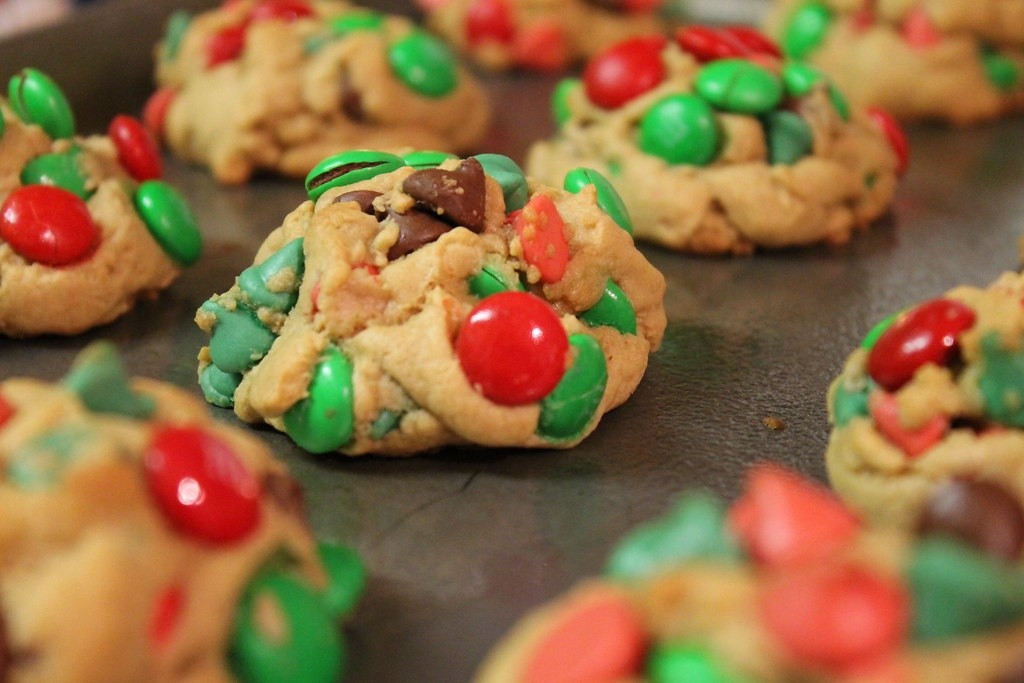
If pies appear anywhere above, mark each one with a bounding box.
[0,0,1024,683]
